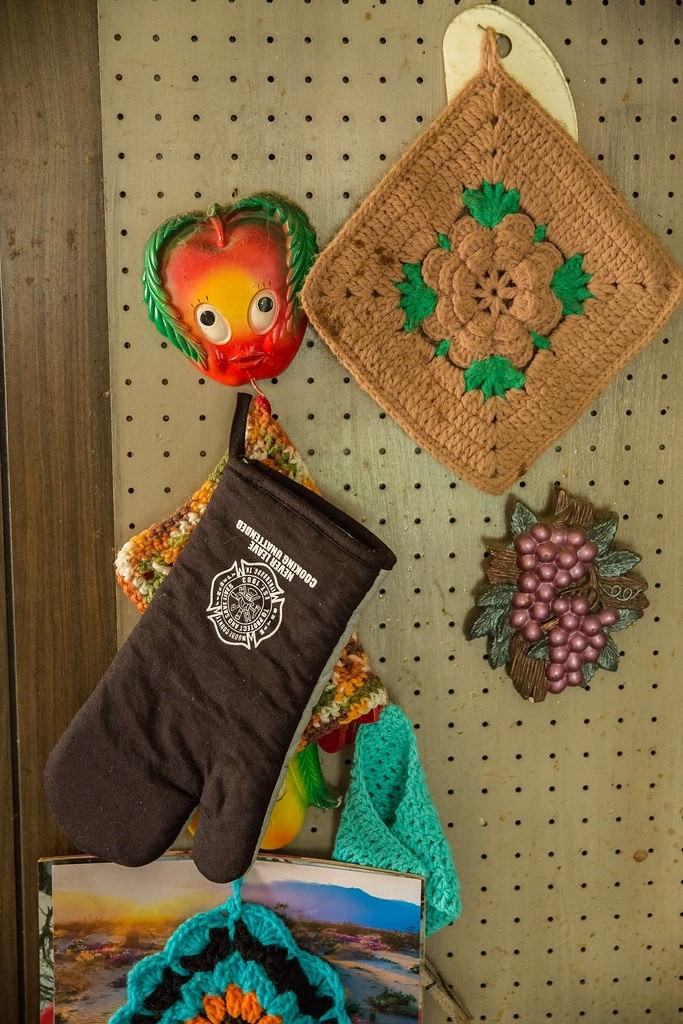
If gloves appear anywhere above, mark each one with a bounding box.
[40,455,398,884]
